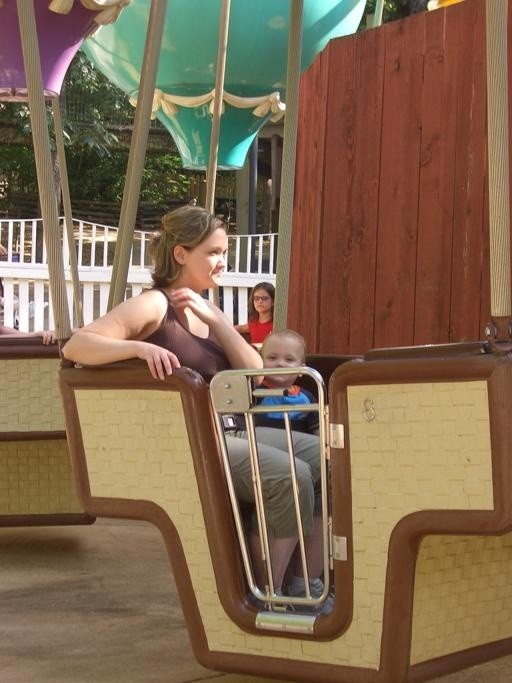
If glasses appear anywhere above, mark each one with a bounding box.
[254,295,272,301]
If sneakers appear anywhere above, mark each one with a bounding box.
[282,578,335,613]
[248,586,295,612]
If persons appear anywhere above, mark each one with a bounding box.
[63,206,340,615]
[0,322,79,345]
[256,328,323,435]
[232,281,276,347]
